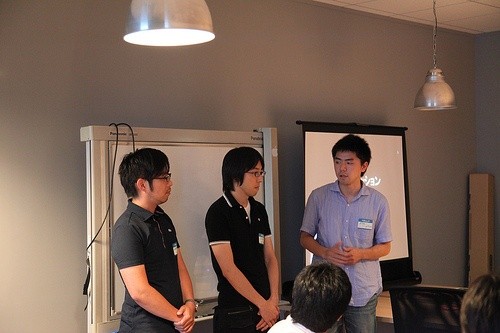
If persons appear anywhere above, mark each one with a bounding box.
[299,135,393,333]
[267,261,352,333]
[111,148,199,333]
[204,147,280,333]
[459,274,500,333]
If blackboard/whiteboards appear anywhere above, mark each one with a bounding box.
[81,125,285,333]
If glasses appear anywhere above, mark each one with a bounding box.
[153,173,172,181]
[246,171,266,177]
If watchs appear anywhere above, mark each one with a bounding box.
[185,299,199,312]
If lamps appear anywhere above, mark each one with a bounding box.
[413,0,459,112]
[122,0,215,47]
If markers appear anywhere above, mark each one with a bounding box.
[195,314,214,319]
